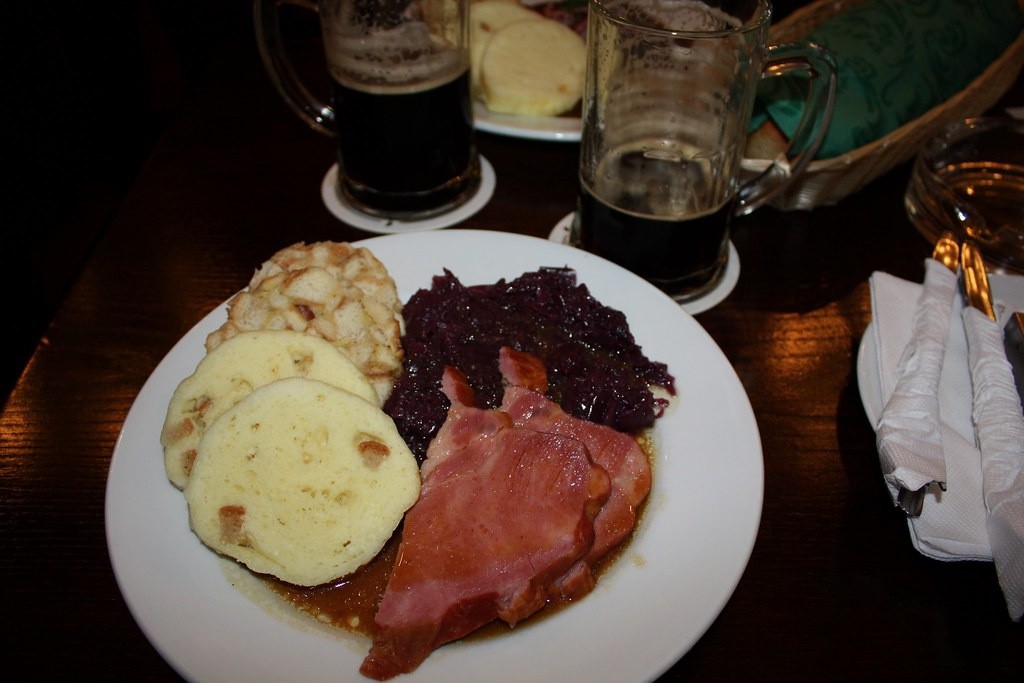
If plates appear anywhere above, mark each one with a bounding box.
[466,107,583,155]
[733,0,1024,208]
[104,228,766,683]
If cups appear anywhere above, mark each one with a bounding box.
[567,0,837,308]
[905,118,1024,319]
[248,0,484,220]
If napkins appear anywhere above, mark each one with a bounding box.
[868,272,994,562]
[961,305,1024,622]
[874,260,956,491]
